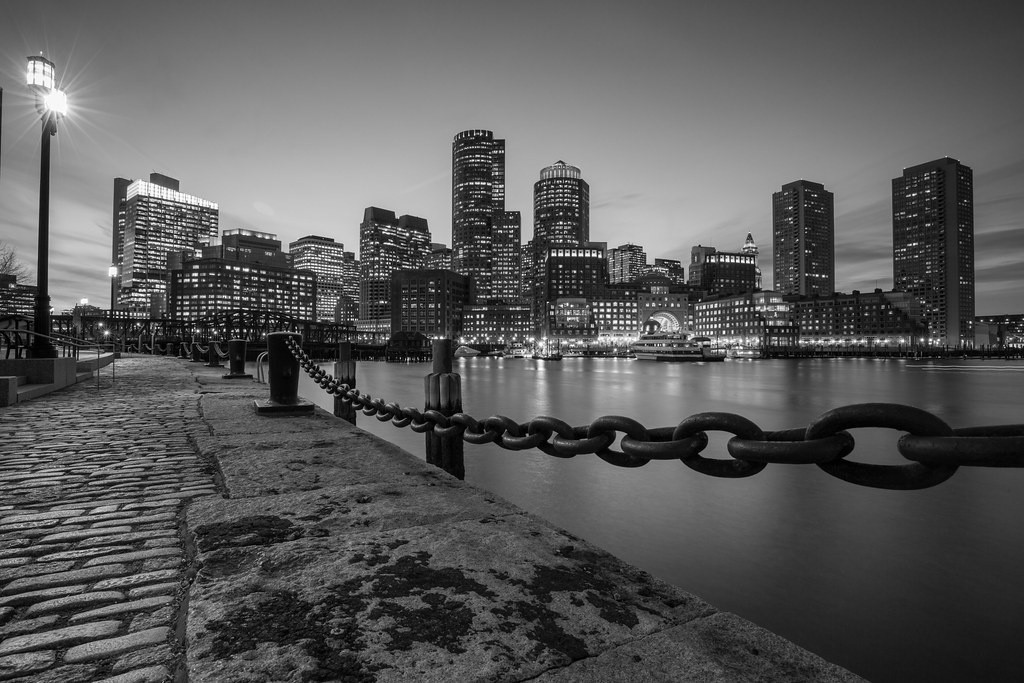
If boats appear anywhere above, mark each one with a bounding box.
[632,332,729,361]
[531,337,563,361]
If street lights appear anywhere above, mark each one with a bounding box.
[26,53,69,357]
